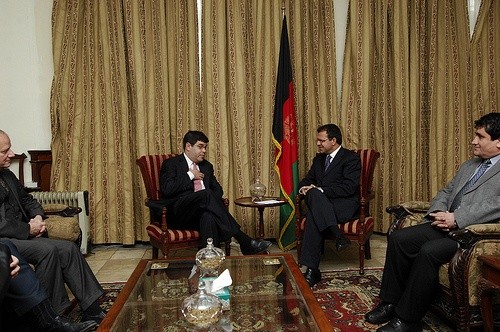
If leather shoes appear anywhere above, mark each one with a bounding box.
[241,239,272,251]
[304,267,322,286]
[82,310,107,322]
[53,316,97,332]
[376,320,423,332]
[336,237,351,250]
[365,301,400,324]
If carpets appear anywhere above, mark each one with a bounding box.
[63,267,459,332]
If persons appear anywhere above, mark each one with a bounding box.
[0,235,98,332]
[158,131,274,257]
[0,128,108,328]
[364,112,500,332]
[296,123,362,291]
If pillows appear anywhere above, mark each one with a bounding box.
[44,217,81,241]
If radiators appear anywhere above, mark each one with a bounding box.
[28,191,78,218]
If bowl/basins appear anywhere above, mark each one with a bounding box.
[194,237,227,273]
[181,280,224,329]
[249,177,267,201]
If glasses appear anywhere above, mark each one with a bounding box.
[319,138,330,144]
[193,144,208,150]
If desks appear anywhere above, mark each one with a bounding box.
[96,253,335,332]
[234,197,288,255]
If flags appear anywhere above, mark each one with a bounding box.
[273,14,304,253]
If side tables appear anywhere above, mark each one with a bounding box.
[477,254,500,332]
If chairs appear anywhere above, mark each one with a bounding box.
[386,202,500,332]
[296,149,389,270]
[9,153,27,187]
[136,154,230,259]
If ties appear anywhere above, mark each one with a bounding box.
[325,156,332,171]
[192,162,202,192]
[449,159,492,212]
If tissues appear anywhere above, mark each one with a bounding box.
[198,268,233,311]
